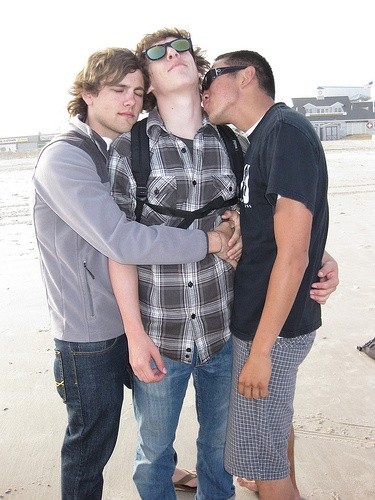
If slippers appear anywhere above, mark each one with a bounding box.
[174,469,198,493]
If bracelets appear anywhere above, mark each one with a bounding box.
[214,229,224,256]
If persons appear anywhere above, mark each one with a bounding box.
[201,50,330,500]
[30,48,243,499]
[107,28,339,500]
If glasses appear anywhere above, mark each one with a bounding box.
[141,38,191,61]
[202,65,246,95]
